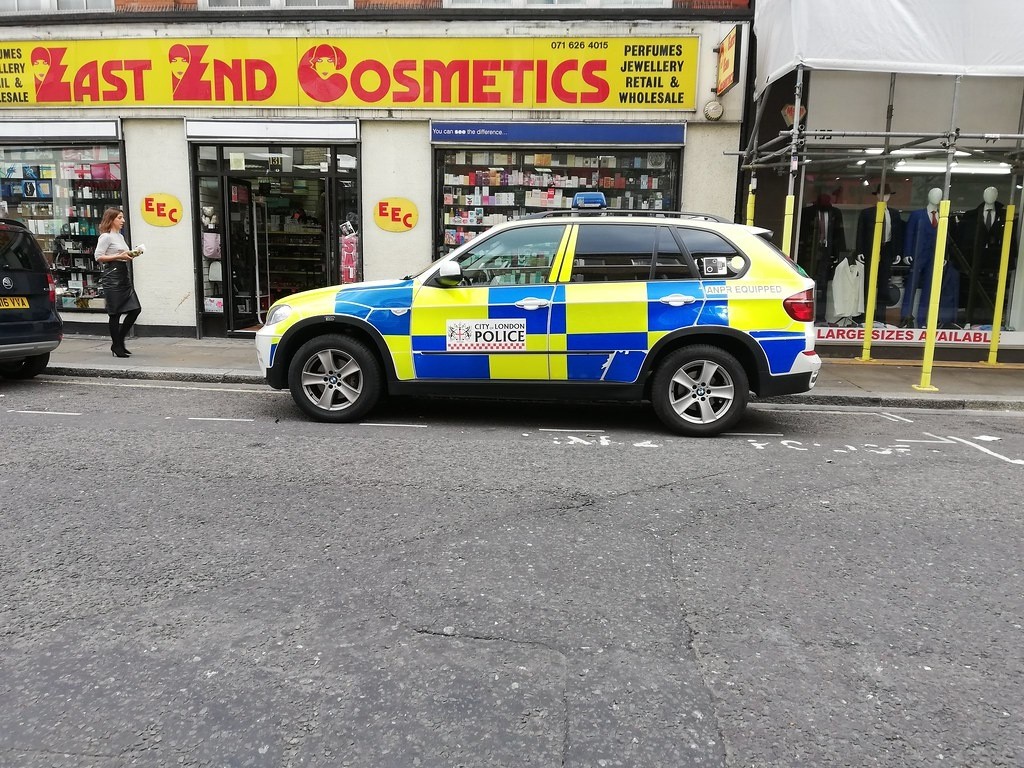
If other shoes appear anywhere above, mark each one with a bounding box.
[897,314,915,328]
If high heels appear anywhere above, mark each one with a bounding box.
[111,340,131,358]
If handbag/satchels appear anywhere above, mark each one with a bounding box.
[203,233,224,313]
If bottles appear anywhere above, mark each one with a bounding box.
[449,208,454,225]
[455,209,460,223]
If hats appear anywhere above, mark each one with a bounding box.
[813,193,832,211]
[872,184,896,195]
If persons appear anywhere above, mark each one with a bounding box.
[94,207,141,358]
[796,183,1017,329]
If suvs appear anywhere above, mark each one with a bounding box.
[255,192,824,438]
[0,217,63,386]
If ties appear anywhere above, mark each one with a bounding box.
[931,211,937,229]
[882,213,886,246]
[818,211,825,241]
[986,209,993,232]
